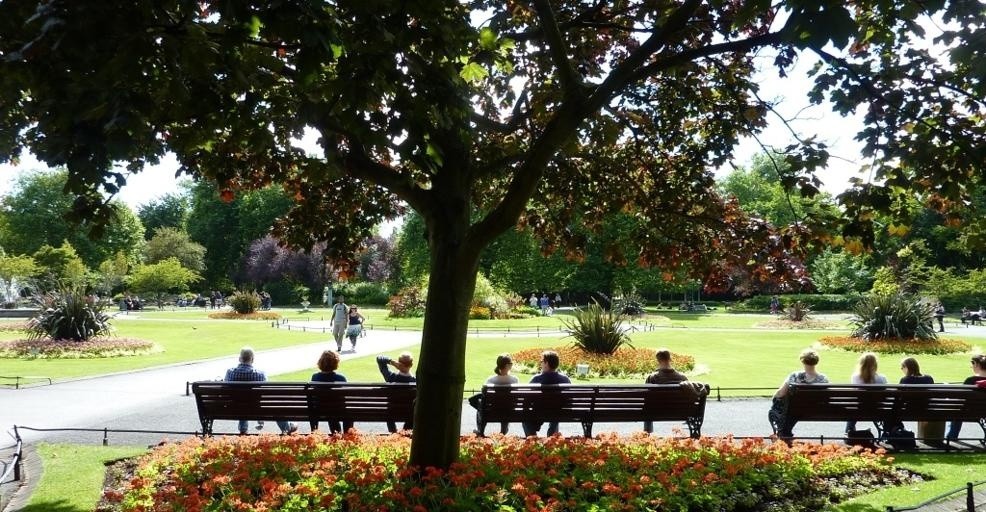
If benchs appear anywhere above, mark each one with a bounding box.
[190,377,414,439]
[765,379,986,446]
[474,383,708,441]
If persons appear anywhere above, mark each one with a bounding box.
[524,353,570,436]
[656,296,780,315]
[529,293,538,306]
[948,354,986,442]
[540,294,549,316]
[224,350,297,434]
[375,353,415,433]
[40,287,274,310]
[311,350,355,436]
[846,354,886,437]
[328,292,348,354]
[344,305,364,350]
[923,301,985,334]
[644,350,688,434]
[472,356,520,433]
[555,292,561,309]
[893,358,934,414]
[775,350,827,415]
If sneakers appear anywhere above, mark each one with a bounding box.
[282,422,297,435]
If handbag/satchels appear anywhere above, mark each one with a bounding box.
[885,430,916,450]
[848,429,876,452]
